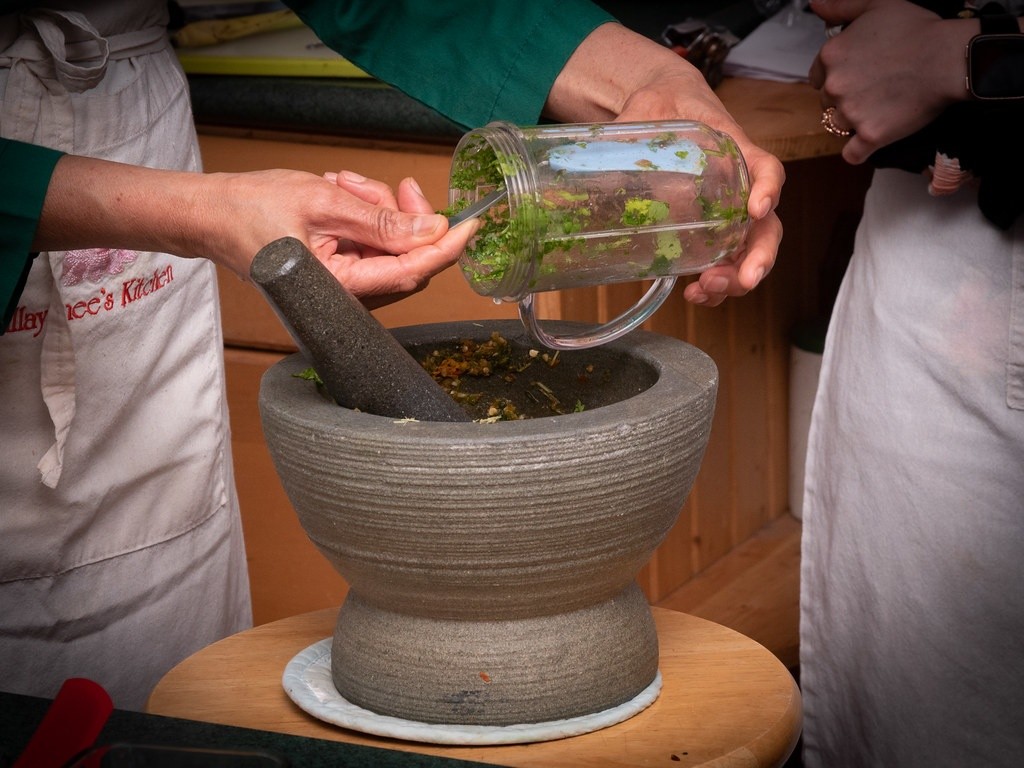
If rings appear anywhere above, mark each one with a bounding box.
[821,107,851,137]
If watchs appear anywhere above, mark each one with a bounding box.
[964,2,1024,123]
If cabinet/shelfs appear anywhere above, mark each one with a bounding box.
[202,76,841,658]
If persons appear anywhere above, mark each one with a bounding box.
[0,0,786,714]
[797,0,1024,768]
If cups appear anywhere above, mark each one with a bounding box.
[449,123,753,350]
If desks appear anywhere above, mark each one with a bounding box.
[145,600,804,768]
